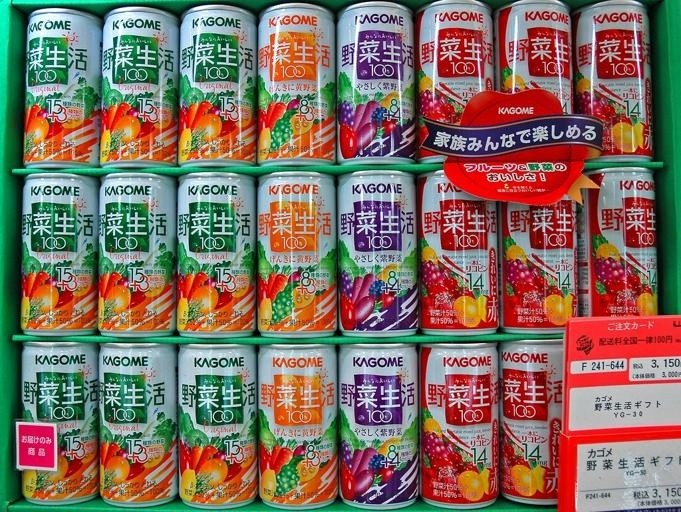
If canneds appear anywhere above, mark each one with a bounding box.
[176,2,258,172]
[500,340,565,507]
[178,345,258,510]
[421,343,501,510]
[17,342,100,507]
[258,3,337,165]
[99,6,179,169]
[22,8,102,170]
[100,343,179,508]
[418,172,499,334]
[18,174,99,337]
[258,170,338,340]
[500,193,579,334]
[178,172,258,338]
[573,3,656,164]
[495,0,577,119]
[417,1,497,165]
[100,171,178,338]
[579,167,659,319]
[337,344,421,511]
[338,170,419,336]
[337,1,418,167]
[258,345,337,511]
